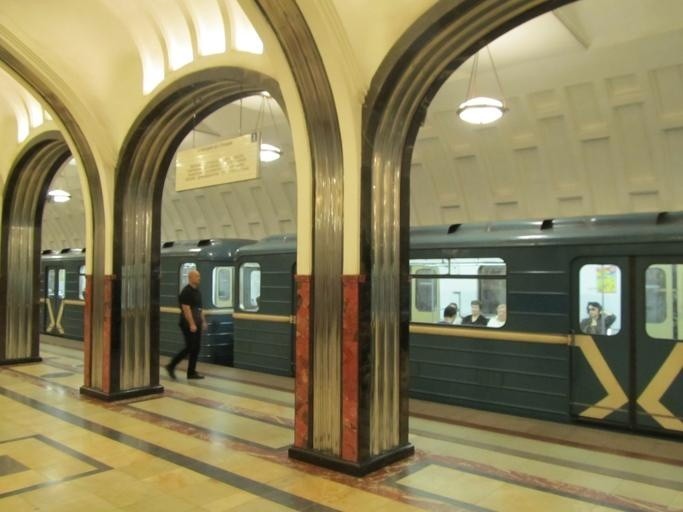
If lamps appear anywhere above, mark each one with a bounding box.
[455,40,509,126]
[250,89,282,162]
[47,189,72,203]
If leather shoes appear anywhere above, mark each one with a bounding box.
[166,364,204,378]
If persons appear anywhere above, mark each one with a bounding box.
[487,303,507,328]
[461,301,487,327]
[437,306,458,325]
[164,270,208,379]
[579,302,616,336]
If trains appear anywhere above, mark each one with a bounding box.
[39,210,683,441]
